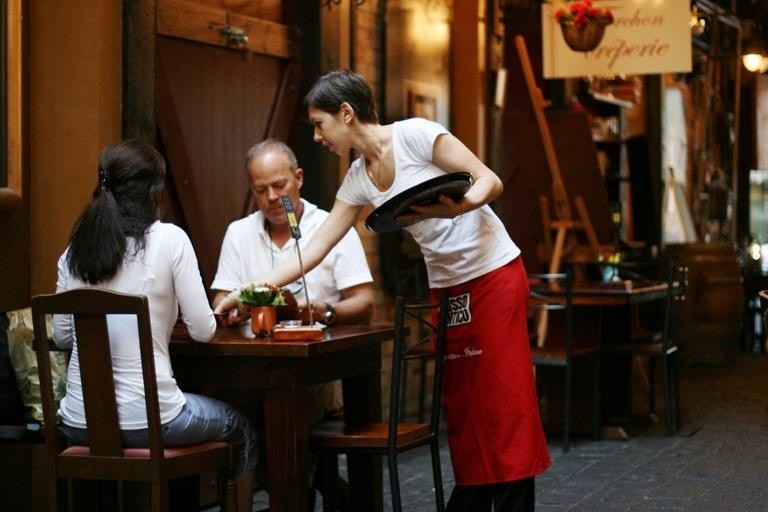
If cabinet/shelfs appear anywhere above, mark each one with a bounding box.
[574,93,627,244]
[682,0,743,241]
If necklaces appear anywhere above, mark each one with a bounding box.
[366,134,382,192]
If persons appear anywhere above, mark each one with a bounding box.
[209,138,374,512]
[213,70,552,512]
[52,137,268,512]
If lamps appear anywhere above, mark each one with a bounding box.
[742,38,768,74]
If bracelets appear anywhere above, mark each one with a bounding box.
[451,202,462,226]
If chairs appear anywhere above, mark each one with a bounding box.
[526,271,600,450]
[622,268,686,436]
[31,287,238,512]
[309,294,450,512]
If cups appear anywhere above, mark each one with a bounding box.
[252,306,276,337]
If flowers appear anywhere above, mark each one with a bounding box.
[555,0,615,29]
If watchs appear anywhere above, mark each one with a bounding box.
[322,301,337,325]
